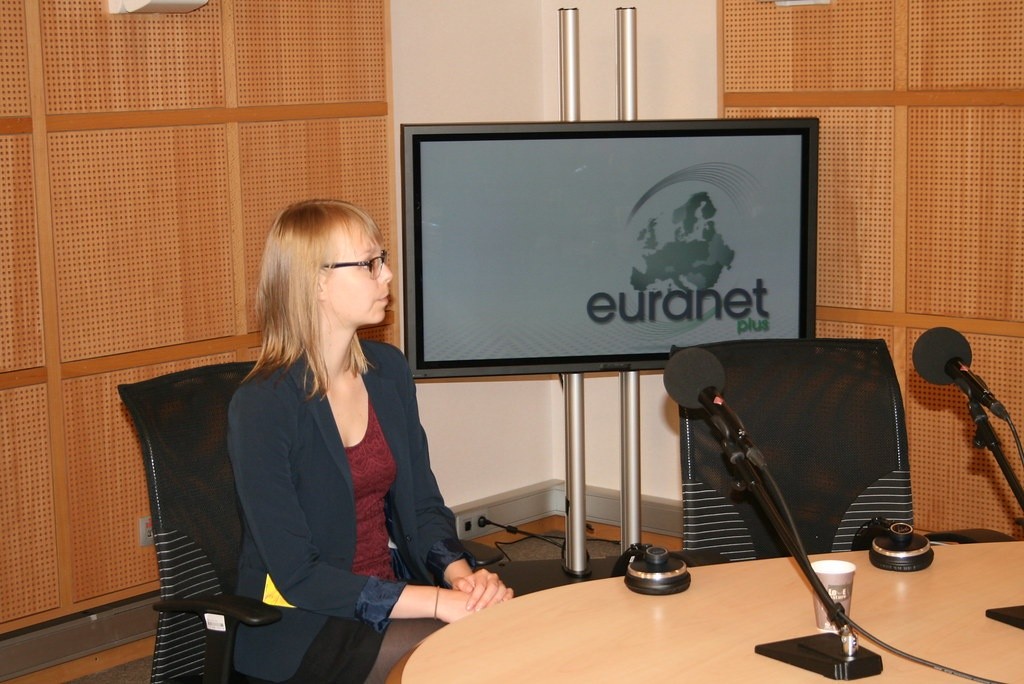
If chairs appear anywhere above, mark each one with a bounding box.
[674,334,918,567]
[117,358,280,681]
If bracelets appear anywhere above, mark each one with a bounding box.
[434,585,441,620]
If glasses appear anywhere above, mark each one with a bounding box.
[318,249,389,281]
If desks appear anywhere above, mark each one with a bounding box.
[391,530,1024,683]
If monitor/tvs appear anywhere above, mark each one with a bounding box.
[402,118,823,380]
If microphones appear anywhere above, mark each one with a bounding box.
[911,326,1011,422]
[662,347,768,469]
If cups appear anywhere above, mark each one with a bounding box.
[812,559,856,632]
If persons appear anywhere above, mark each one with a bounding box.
[226,198,515,684]
